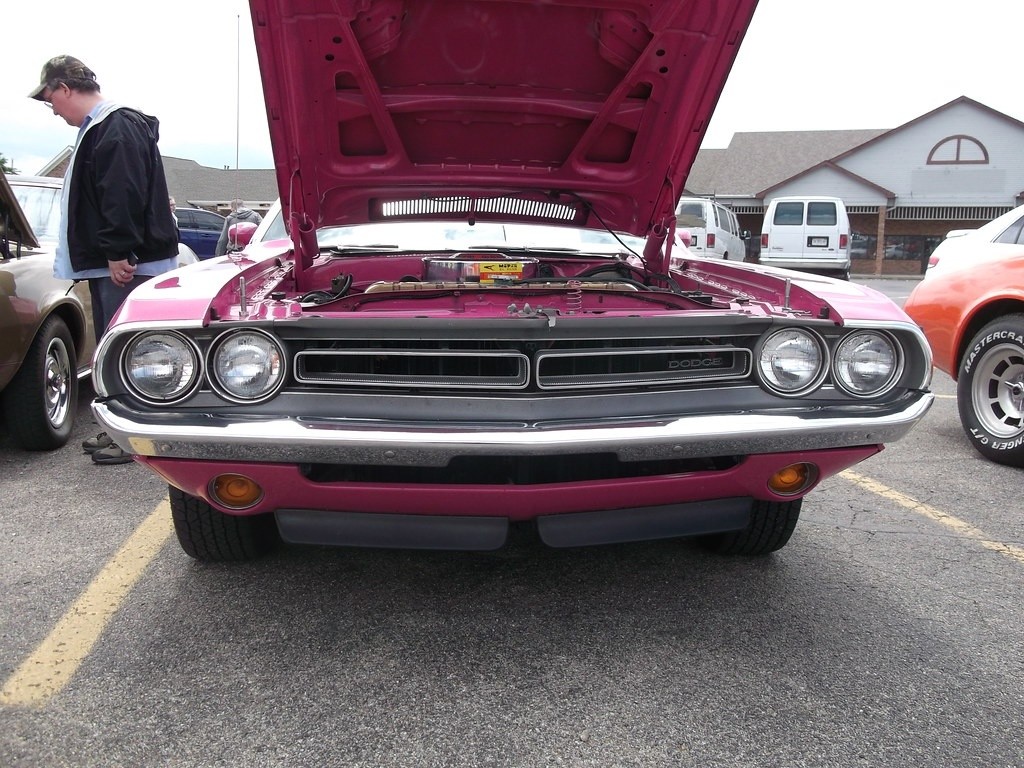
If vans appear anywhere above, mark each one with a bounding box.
[758,195,850,282]
[675,198,751,263]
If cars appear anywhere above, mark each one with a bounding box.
[90,0,934,560]
[850,240,910,261]
[902,202,1024,467]
[0,163,200,452]
[174,207,227,262]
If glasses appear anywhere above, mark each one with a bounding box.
[44,81,61,110]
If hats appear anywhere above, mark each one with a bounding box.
[26,54,96,99]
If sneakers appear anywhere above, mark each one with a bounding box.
[82,421,115,453]
[91,441,136,464]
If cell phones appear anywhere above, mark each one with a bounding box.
[128,250,139,267]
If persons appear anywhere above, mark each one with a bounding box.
[215,199,262,257]
[26,55,179,465]
[169,196,180,241]
